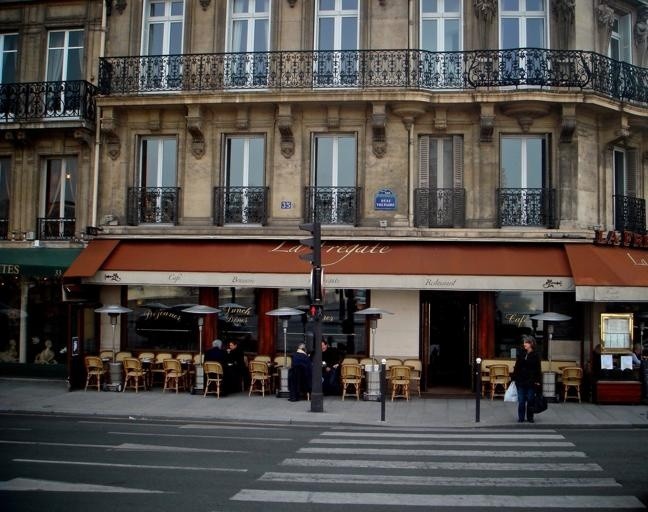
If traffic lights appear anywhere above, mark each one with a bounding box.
[310,305,324,319]
[299,222,321,267]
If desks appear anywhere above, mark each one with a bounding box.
[481,369,514,376]
[541,369,564,382]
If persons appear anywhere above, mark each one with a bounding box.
[1,340,18,362]
[511,335,541,423]
[322,341,340,395]
[633,343,644,365]
[290,343,311,401]
[38,340,58,365]
[226,341,245,371]
[203,339,230,396]
[591,345,601,370]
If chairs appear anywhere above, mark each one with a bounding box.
[203,361,224,399]
[383,357,403,393]
[341,357,358,367]
[253,354,293,394]
[83,355,105,392]
[360,357,378,370]
[402,358,423,398]
[388,364,412,403]
[246,360,269,399]
[83,349,207,391]
[560,367,584,403]
[122,357,146,394]
[161,359,186,394]
[340,363,362,402]
[489,364,510,401]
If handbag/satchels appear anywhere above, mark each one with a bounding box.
[530,384,547,413]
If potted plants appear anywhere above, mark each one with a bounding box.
[591,367,642,404]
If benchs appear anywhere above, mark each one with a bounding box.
[480,359,577,398]
